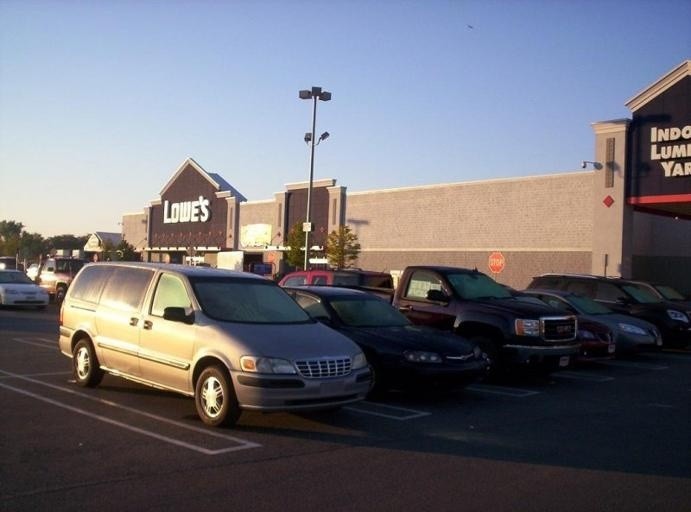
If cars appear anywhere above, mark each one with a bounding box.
[0,254,89,310]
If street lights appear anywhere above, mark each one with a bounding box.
[299,86,331,271]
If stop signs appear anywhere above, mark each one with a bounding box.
[488,252,506,274]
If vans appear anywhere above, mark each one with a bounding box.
[55,259,372,430]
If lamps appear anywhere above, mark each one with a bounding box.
[581,160,603,169]
